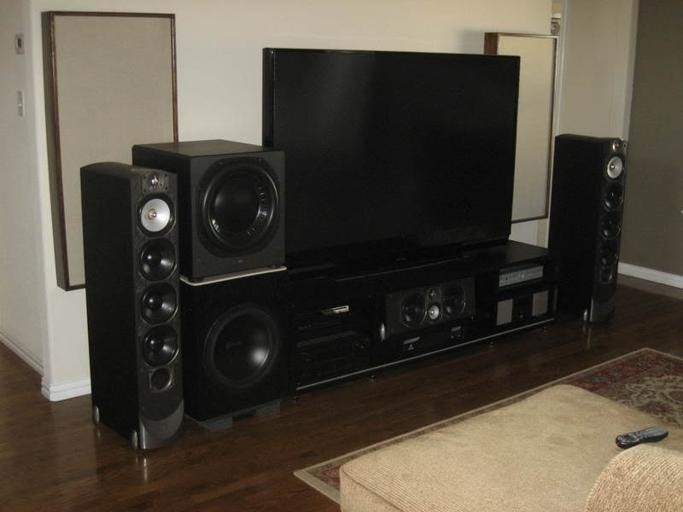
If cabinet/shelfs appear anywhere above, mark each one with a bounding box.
[287,237,558,405]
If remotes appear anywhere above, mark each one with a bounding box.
[616,425,669,451]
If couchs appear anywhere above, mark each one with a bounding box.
[331,380,682,512]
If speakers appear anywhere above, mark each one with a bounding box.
[549,133,631,326]
[180,266,293,417]
[132,137,286,283]
[379,274,479,340]
[79,160,185,453]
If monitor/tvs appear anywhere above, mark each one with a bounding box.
[262,45,521,284]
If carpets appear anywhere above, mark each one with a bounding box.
[288,347,682,505]
